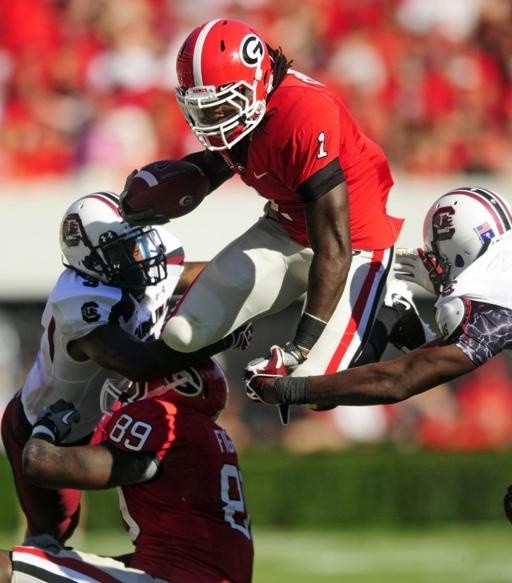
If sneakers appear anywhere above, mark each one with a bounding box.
[385,282,435,355]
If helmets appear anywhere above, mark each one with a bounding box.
[59,195,166,286]
[99,357,227,424]
[423,188,511,283]
[173,20,272,151]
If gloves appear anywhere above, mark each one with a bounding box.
[394,250,447,294]
[32,400,79,442]
[118,171,168,226]
[223,322,255,351]
[242,343,303,382]
[246,346,290,405]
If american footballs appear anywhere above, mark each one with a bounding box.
[121,159,206,217]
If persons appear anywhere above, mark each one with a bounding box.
[0,358,254,583]
[1,192,255,548]
[119,17,407,412]
[244,186,512,405]
